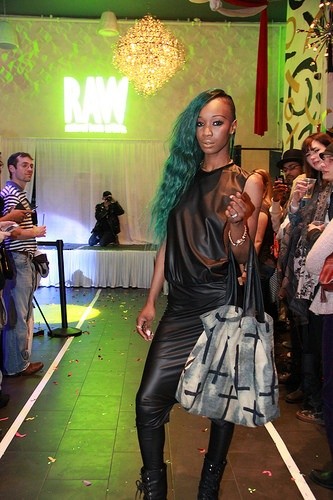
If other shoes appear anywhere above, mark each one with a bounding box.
[113,235,119,246]
[281,339,291,364]
[295,408,325,426]
[0,394,10,408]
[278,371,291,382]
[285,391,309,403]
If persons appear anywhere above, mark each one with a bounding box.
[249,149,305,381]
[287,125,333,488]
[89,190,124,247]
[135,89,264,500]
[0,150,18,405]
[0,152,46,376]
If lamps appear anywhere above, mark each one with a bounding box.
[97,9,119,36]
[0,17,19,48]
[112,13,188,98]
[295,0,333,72]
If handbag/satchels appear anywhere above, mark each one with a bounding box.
[174,241,281,428]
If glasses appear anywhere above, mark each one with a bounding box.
[282,164,302,172]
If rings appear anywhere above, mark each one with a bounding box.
[232,213,237,219]
[136,326,142,329]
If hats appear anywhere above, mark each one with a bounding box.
[319,141,333,160]
[276,149,305,169]
[102,191,112,197]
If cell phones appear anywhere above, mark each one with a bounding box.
[4,223,19,232]
[275,175,284,184]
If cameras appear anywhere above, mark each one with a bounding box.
[105,197,112,201]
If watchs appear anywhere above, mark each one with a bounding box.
[228,224,247,247]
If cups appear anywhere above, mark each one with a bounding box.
[302,178,316,198]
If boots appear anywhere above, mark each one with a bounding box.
[197,453,227,500]
[133,462,167,500]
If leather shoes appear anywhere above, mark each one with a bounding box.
[7,361,44,377]
[308,468,333,490]
